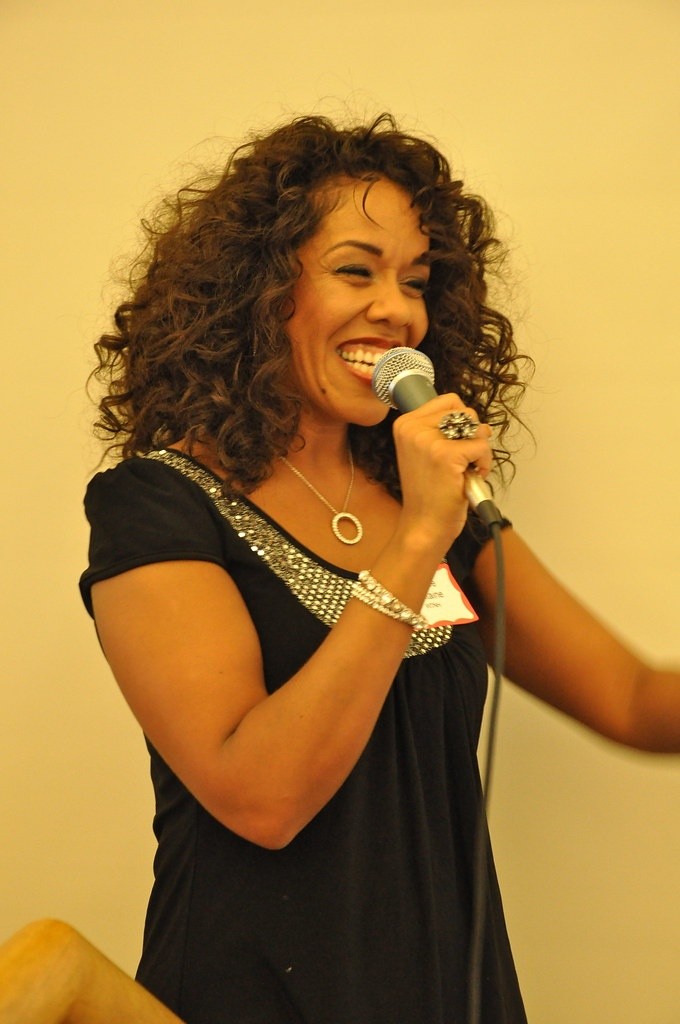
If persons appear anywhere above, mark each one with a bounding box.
[79,113,680,1024]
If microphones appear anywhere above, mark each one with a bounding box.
[372,346,503,530]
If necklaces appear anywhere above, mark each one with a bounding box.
[265,438,363,545]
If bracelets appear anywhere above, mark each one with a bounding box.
[347,571,429,633]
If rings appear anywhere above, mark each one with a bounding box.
[435,411,481,440]
